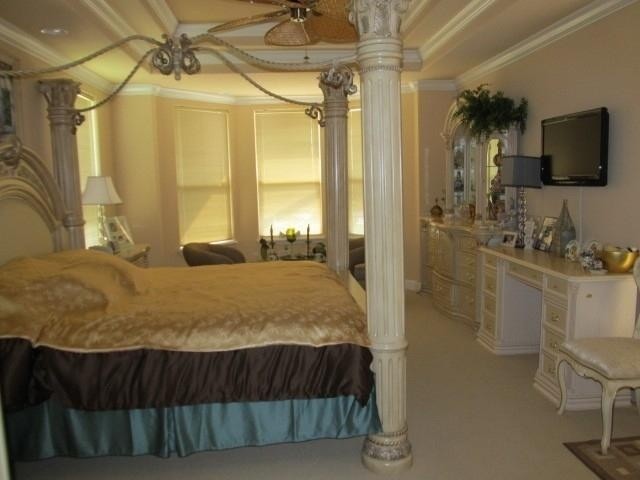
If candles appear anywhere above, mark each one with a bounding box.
[270,225,275,248]
[306,225,310,255]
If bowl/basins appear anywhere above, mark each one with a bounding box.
[601,247,639,274]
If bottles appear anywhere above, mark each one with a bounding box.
[430,196,443,217]
[555,199,576,257]
[454,149,464,192]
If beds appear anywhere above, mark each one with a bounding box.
[0,137,380,460]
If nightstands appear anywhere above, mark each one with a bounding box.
[90,243,150,267]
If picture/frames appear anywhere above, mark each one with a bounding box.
[502,231,517,246]
[535,217,558,252]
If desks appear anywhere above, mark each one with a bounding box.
[477,245,639,410]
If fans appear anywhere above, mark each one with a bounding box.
[207,0,359,45]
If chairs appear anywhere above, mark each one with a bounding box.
[555,315,639,454]
[183,243,245,266]
[350,236,365,288]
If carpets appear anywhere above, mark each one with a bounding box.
[563,437,640,480]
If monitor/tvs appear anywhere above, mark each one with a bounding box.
[541,107,609,187]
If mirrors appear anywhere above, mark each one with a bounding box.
[441,98,518,225]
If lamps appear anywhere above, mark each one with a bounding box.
[500,156,541,247]
[81,175,123,254]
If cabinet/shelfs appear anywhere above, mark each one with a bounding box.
[423,218,517,330]
